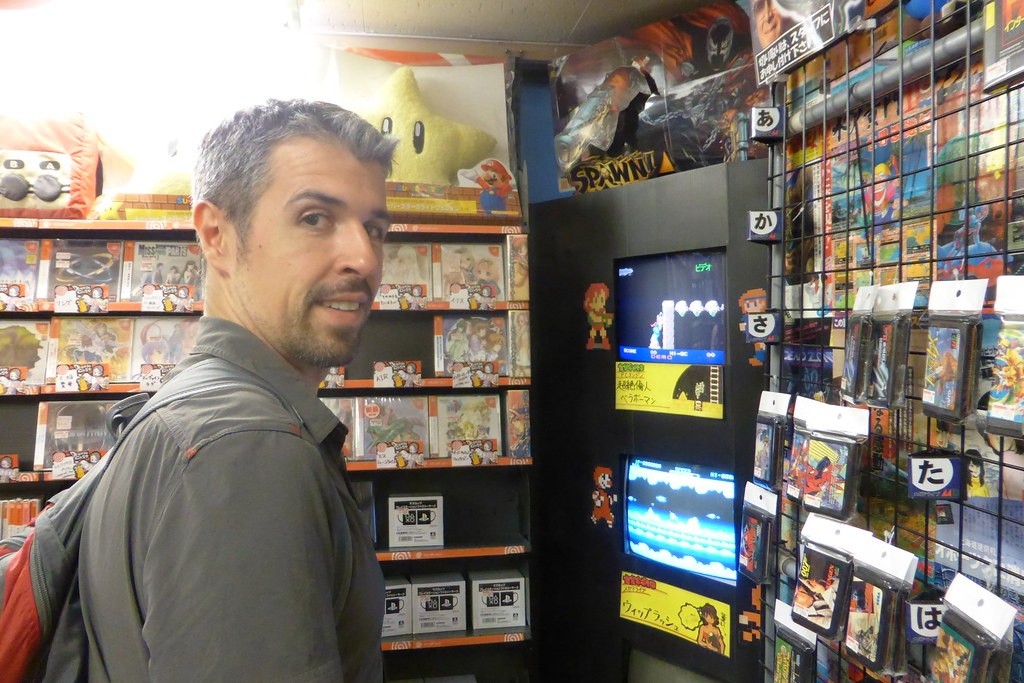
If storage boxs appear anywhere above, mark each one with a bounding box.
[0,235,529,642]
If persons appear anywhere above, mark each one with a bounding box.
[751,1,783,49]
[79,99,394,683]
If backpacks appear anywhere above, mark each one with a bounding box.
[0,358,306,683]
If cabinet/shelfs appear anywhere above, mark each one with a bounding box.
[1,216,537,653]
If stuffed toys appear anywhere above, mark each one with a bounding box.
[362,68,497,185]
[0,117,134,217]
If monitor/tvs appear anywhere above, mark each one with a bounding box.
[623,455,739,587]
[613,246,727,367]
